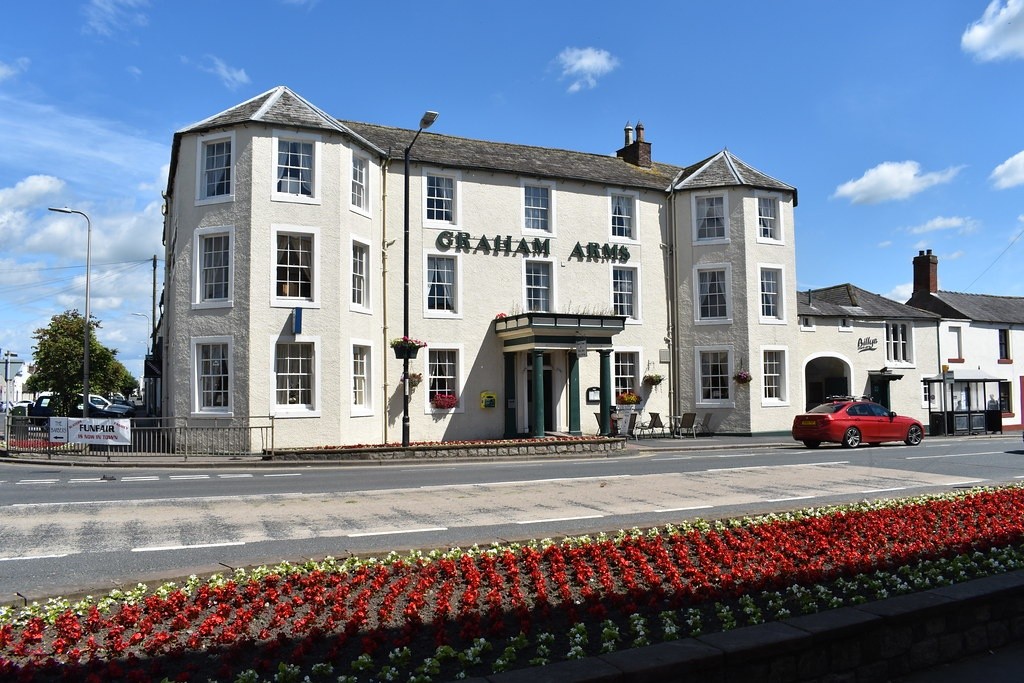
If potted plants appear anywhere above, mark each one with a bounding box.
[401,373,422,387]
[643,374,664,385]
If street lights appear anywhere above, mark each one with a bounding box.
[402,110,441,447]
[47,206,92,418]
[131,312,150,415]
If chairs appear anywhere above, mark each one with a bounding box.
[593,411,713,442]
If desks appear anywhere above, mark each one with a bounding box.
[666,415,683,438]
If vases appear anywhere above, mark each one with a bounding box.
[435,403,454,408]
[737,379,747,384]
[617,399,632,404]
[394,346,419,359]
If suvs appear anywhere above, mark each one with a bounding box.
[0,391,135,433]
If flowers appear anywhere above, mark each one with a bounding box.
[496,313,505,319]
[434,393,457,408]
[617,393,641,403]
[732,369,752,382]
[391,337,427,349]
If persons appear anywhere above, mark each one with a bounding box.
[988,395,999,434]
[955,400,966,411]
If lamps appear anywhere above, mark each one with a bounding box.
[407,111,438,152]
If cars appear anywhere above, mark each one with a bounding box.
[791,401,925,448]
